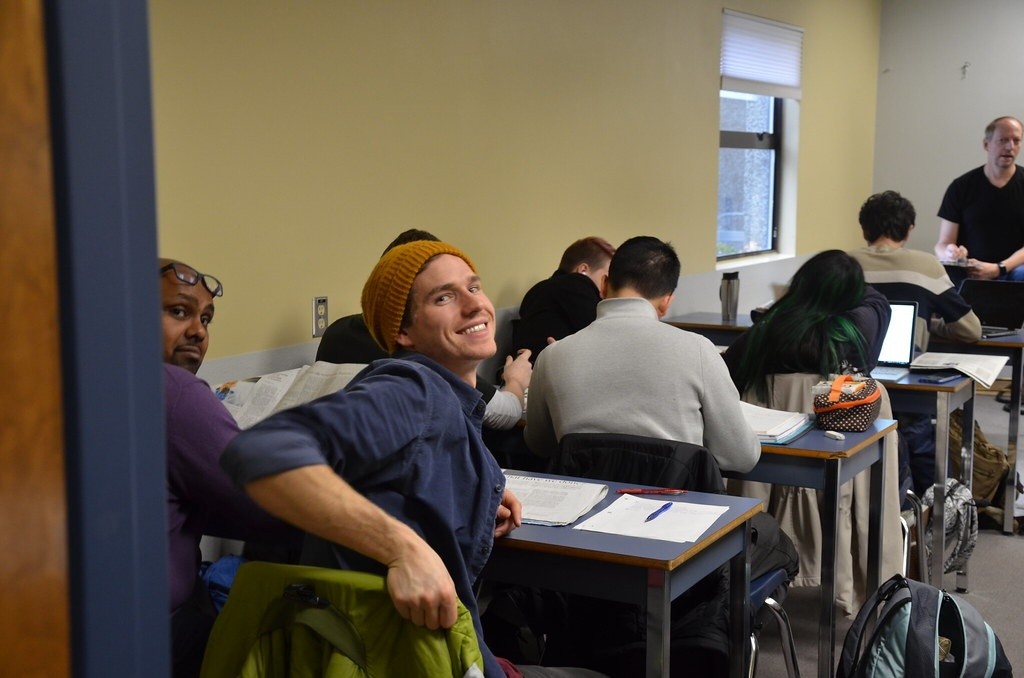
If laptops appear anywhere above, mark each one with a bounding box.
[957,278,1024,338]
[870,301,918,382]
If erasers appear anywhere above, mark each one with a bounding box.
[824,430,845,440]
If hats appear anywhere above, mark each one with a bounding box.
[361,240,478,356]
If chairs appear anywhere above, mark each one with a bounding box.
[171,278,1024,678]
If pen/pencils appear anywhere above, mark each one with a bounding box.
[499,353,520,389]
[645,502,673,522]
[616,489,687,496]
[947,248,952,253]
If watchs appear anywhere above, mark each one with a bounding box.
[998,262,1006,277]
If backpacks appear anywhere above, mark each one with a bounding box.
[836,574,1013,678]
[922,478,979,577]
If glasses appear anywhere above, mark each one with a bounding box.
[161,264,223,297]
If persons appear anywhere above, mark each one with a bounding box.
[933,116,1024,283]
[221,239,610,678]
[315,228,533,432]
[847,189,983,502]
[160,257,245,678]
[527,237,616,374]
[724,250,910,494]
[527,237,800,678]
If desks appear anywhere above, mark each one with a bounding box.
[493,383,898,678]
[202,467,764,678]
[662,310,1024,533]
[870,362,975,594]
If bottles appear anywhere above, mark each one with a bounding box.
[720,271,740,322]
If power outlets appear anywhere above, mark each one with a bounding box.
[312,296,329,337]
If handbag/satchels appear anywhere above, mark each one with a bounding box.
[813,373,882,432]
[950,407,1009,506]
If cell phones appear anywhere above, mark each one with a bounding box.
[918,373,961,384]
